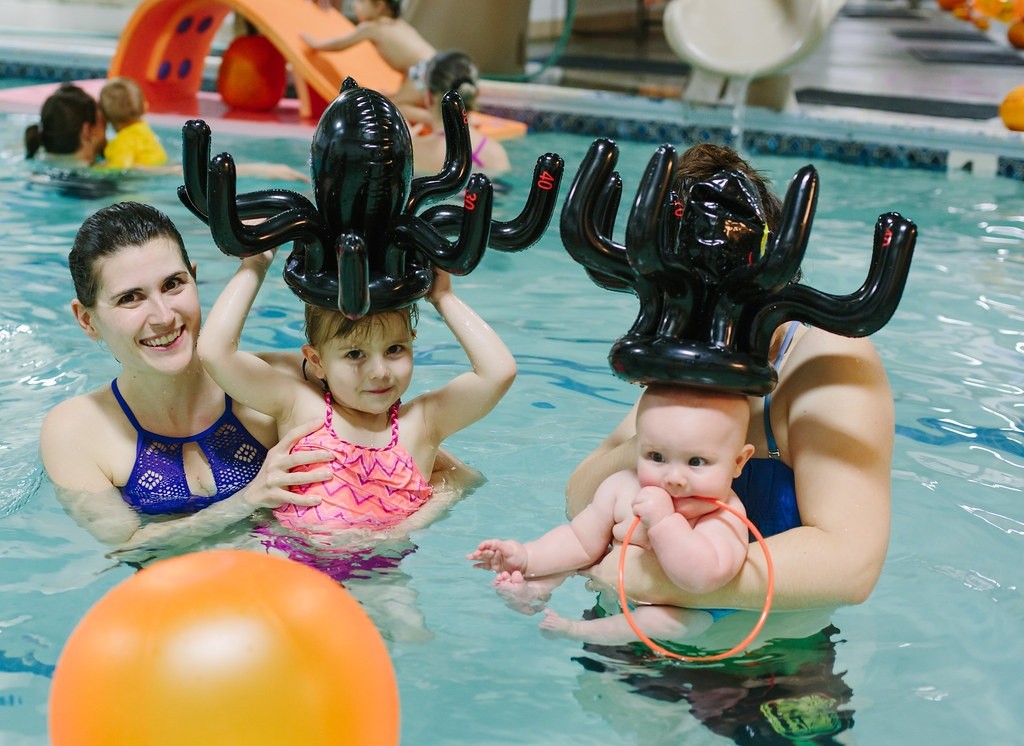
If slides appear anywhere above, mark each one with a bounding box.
[661,0,825,81]
[212,0,529,151]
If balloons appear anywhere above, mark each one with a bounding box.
[50,548,401,746]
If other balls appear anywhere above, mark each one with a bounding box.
[48,549,402,746]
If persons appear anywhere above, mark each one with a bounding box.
[567,144,895,611]
[299,0,438,129]
[465,383,754,597]
[26,85,107,181]
[410,52,512,174]
[39,202,488,514]
[198,218,517,521]
[99,80,167,170]
